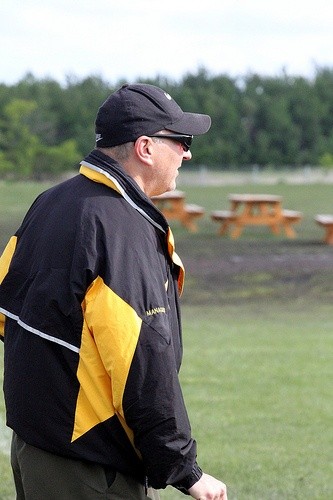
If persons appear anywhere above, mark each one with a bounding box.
[0,83,228,500]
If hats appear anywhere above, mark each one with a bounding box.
[95,83,212,148]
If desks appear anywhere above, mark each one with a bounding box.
[151,191,286,237]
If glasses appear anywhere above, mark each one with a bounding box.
[146,134,194,152]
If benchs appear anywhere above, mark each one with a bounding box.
[151,209,333,247]
